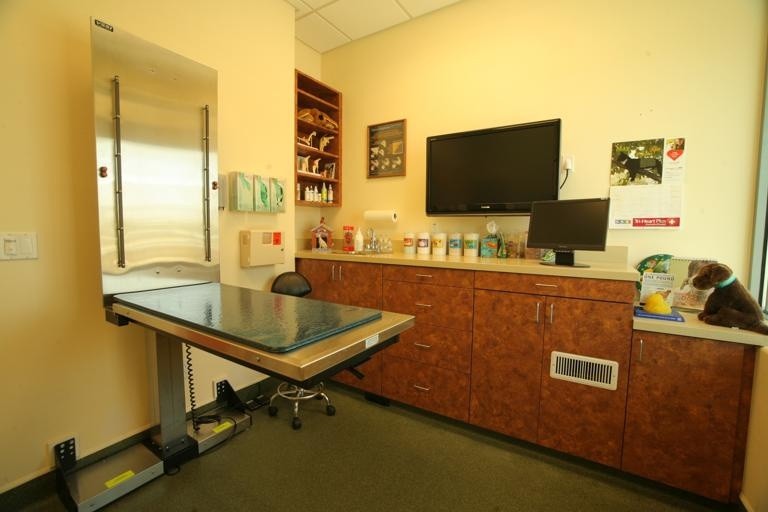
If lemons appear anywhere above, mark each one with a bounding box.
[643,293,664,311]
[648,300,672,315]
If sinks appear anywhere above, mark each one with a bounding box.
[332,251,394,255]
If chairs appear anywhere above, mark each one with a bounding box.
[268,272,335,430]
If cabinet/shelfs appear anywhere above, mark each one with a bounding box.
[296,258,382,397]
[294,69,342,208]
[622,330,757,512]
[382,264,474,426]
[469,270,633,477]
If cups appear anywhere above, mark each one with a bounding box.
[463,232,480,256]
[448,232,462,256]
[402,234,416,256]
[417,233,431,255]
[365,225,393,252]
[431,234,447,257]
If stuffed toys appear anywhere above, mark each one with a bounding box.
[298,106,339,130]
[689,263,768,336]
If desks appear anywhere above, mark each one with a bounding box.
[111,283,415,448]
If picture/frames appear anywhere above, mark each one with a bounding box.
[366,119,405,178]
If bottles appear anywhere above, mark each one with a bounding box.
[354,227,364,252]
[304,182,334,203]
[343,225,354,251]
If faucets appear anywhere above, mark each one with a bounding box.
[367,226,376,250]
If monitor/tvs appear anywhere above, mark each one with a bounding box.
[426,119,560,216]
[526,197,611,267]
